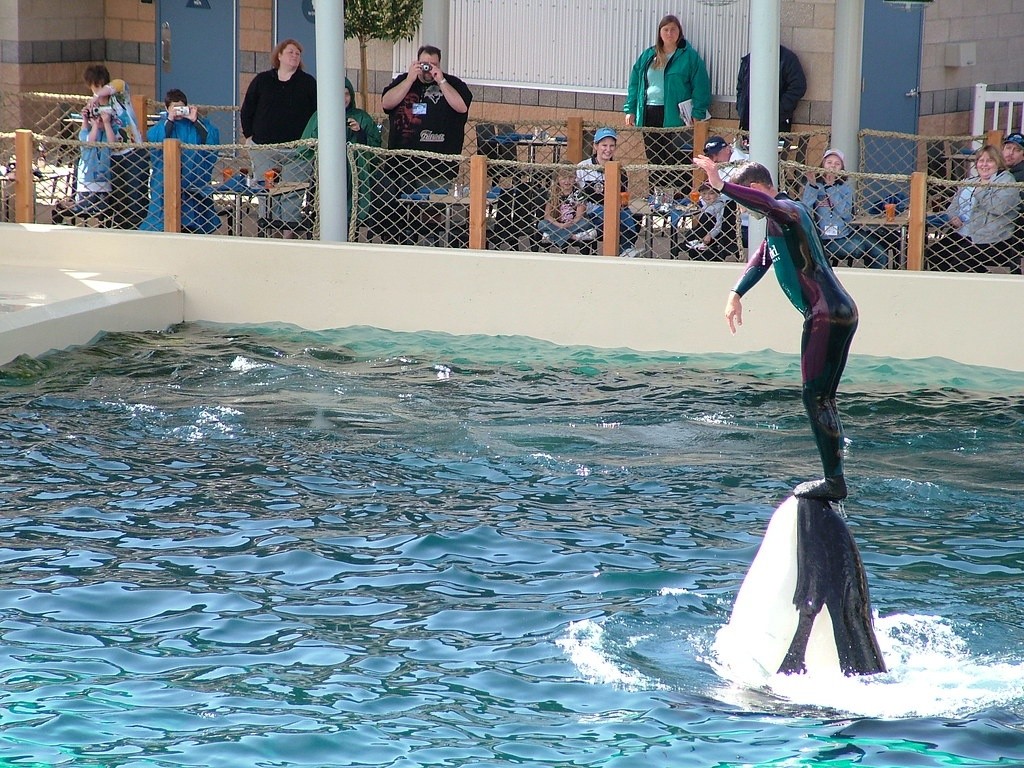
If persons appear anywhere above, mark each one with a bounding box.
[240,39,317,239]
[803,149,889,270]
[1001,132,1024,274]
[576,127,636,255]
[293,77,383,242]
[57,105,116,213]
[735,45,807,182]
[538,161,597,243]
[146,88,222,234]
[623,16,712,199]
[925,145,1019,272]
[673,136,748,261]
[380,46,473,246]
[692,154,858,498]
[82,65,149,230]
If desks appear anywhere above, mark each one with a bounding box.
[485,137,567,182]
[0,167,75,183]
[849,215,952,269]
[186,181,310,237]
[628,198,701,258]
[398,189,499,247]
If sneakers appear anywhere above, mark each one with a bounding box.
[576,229,597,240]
[686,239,707,250]
[542,232,550,243]
[620,248,646,257]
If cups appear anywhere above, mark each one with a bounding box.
[535,126,541,139]
[654,186,674,206]
[453,183,462,198]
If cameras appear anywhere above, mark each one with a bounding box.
[420,62,433,72]
[93,106,112,117]
[174,106,190,116]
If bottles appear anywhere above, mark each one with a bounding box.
[265,166,282,189]
[885,204,896,221]
[223,170,232,183]
[38,150,45,172]
[690,193,699,204]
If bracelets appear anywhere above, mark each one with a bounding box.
[438,78,446,86]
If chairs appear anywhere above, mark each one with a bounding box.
[51,148,153,229]
[367,186,604,256]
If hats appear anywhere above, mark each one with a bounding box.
[698,180,720,194]
[594,128,617,143]
[703,136,728,156]
[1003,133,1024,150]
[822,148,845,165]
